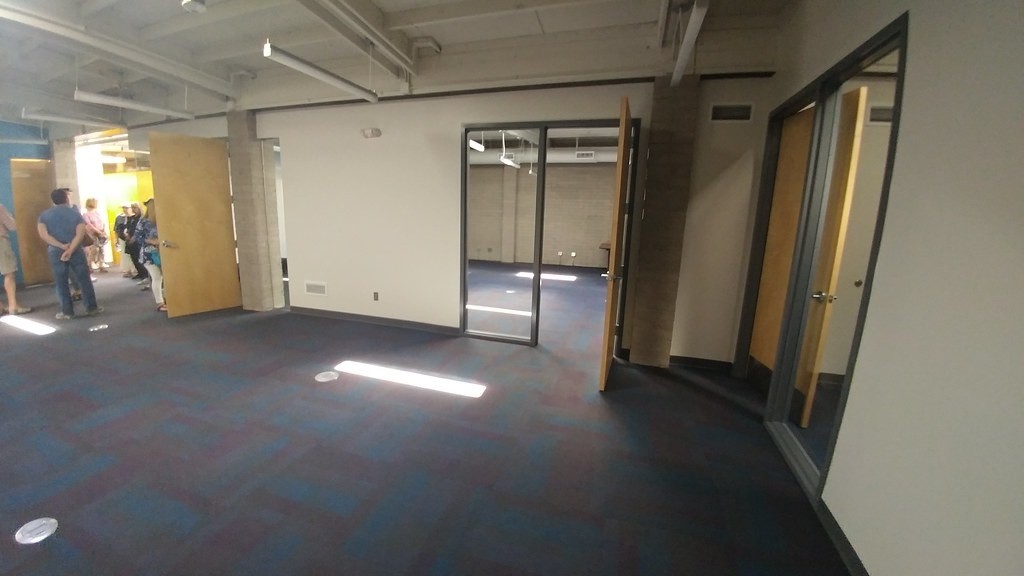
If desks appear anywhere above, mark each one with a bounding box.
[599,243,611,269]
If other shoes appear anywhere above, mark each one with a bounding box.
[72,292,81,300]
[153,302,167,311]
[92,262,98,269]
[132,274,139,279]
[0,308,8,315]
[56,313,74,319]
[85,307,104,315]
[91,277,97,281]
[137,279,149,284]
[140,284,150,291]
[102,263,109,267]
[9,307,31,315]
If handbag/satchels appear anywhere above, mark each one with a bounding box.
[151,250,161,265]
[116,243,122,252]
[82,233,94,248]
[99,230,107,243]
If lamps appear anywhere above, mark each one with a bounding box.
[469,131,485,152]
[500,133,520,169]
[529,143,538,177]
[74,50,195,120]
[263,0,379,104]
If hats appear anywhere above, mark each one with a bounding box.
[144,198,154,205]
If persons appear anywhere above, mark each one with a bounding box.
[112,202,164,291]
[135,199,168,312]
[37,188,104,320]
[81,199,109,270]
[0,203,32,316]
